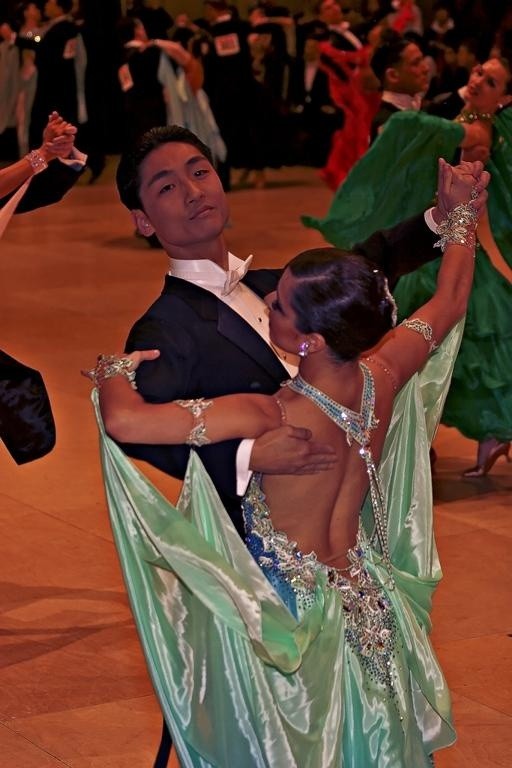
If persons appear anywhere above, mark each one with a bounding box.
[2,108,78,201]
[343,28,472,302]
[1,1,511,195]
[385,49,512,482]
[2,108,91,216]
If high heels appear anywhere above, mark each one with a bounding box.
[462,439,512,478]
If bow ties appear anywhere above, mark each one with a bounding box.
[217,250,256,297]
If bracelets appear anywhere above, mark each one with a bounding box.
[432,202,481,252]
[87,355,140,387]
